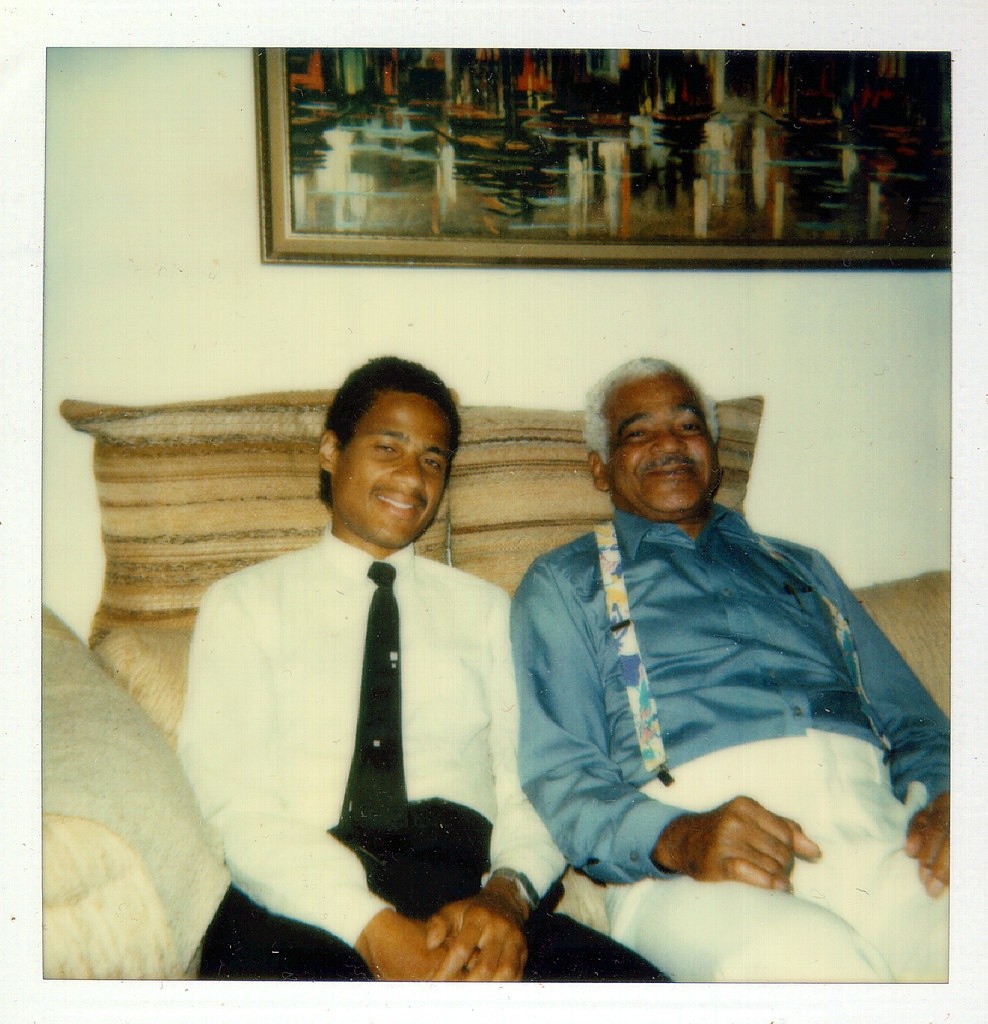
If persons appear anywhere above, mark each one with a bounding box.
[175,356,670,984]
[509,358,950,983]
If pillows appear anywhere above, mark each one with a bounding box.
[60,390,461,749]
[444,397,764,609]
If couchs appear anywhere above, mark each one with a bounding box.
[40,394,949,986]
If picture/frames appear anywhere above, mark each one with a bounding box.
[250,50,951,275]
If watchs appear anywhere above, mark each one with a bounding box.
[493,868,539,909]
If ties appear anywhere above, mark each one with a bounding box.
[337,561,408,895]
